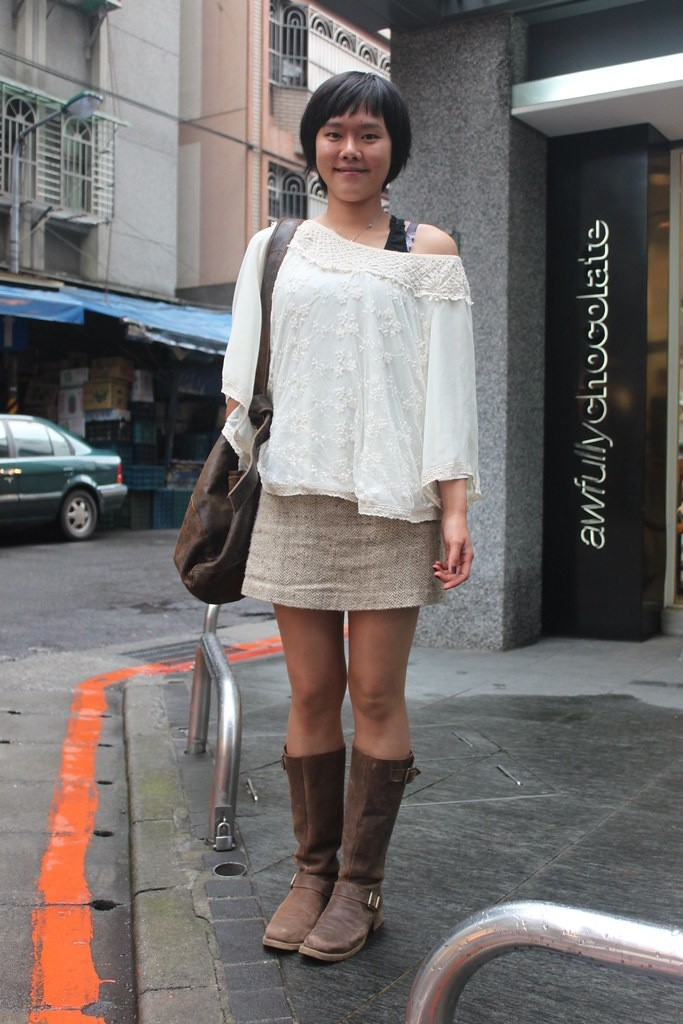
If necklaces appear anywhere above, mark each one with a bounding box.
[328,205,383,241]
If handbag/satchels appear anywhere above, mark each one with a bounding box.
[175,217,306,603]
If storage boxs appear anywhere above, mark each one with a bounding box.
[21,349,226,532]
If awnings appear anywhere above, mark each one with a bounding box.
[0,283,231,355]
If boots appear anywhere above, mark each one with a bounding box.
[262,745,346,951]
[297,743,422,961]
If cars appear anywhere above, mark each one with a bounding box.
[0,413,128,541]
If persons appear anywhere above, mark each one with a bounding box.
[218,72,482,962]
